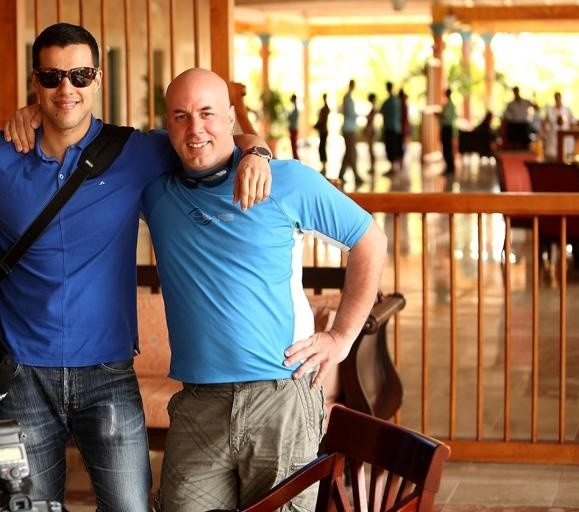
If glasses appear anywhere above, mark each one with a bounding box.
[34,66,99,87]
[176,152,232,188]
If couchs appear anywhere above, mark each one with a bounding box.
[133,261,401,448]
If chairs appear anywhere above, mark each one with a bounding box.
[523,159,579,279]
[246,405,452,512]
[493,149,536,255]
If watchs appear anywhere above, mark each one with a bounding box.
[240,145,272,163]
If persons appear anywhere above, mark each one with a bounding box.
[435,89,457,176]
[3,67,387,512]
[398,90,410,170]
[1,23,273,512]
[471,86,578,160]
[239,85,260,123]
[288,95,299,160]
[339,80,364,184]
[381,82,402,177]
[365,94,377,175]
[261,91,284,159]
[313,94,330,176]
